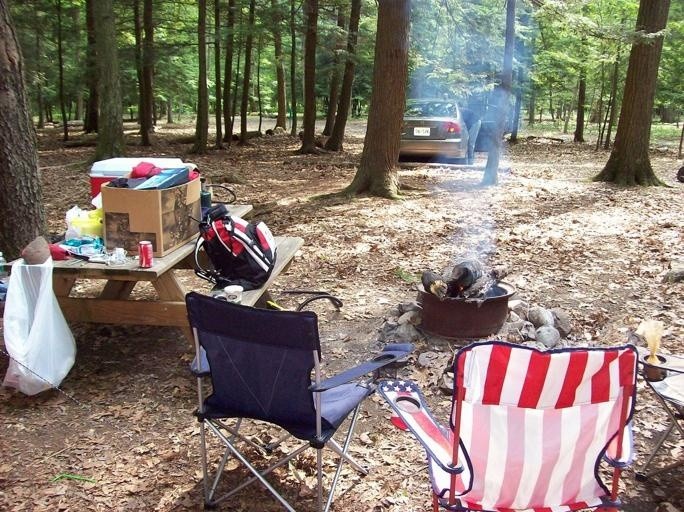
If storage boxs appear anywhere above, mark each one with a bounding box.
[101,174,201,258]
[89,157,183,200]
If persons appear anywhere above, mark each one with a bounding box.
[460,108,482,164]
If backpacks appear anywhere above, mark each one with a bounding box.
[194,213,277,291]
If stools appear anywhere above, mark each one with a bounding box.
[636,374,684,479]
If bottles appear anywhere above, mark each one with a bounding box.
[138,240,153,268]
[201,191,212,218]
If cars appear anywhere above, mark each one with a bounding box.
[400,98,513,164]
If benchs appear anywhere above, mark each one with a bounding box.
[217,235,304,307]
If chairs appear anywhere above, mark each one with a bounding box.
[184,290,372,512]
[379,340,639,512]
